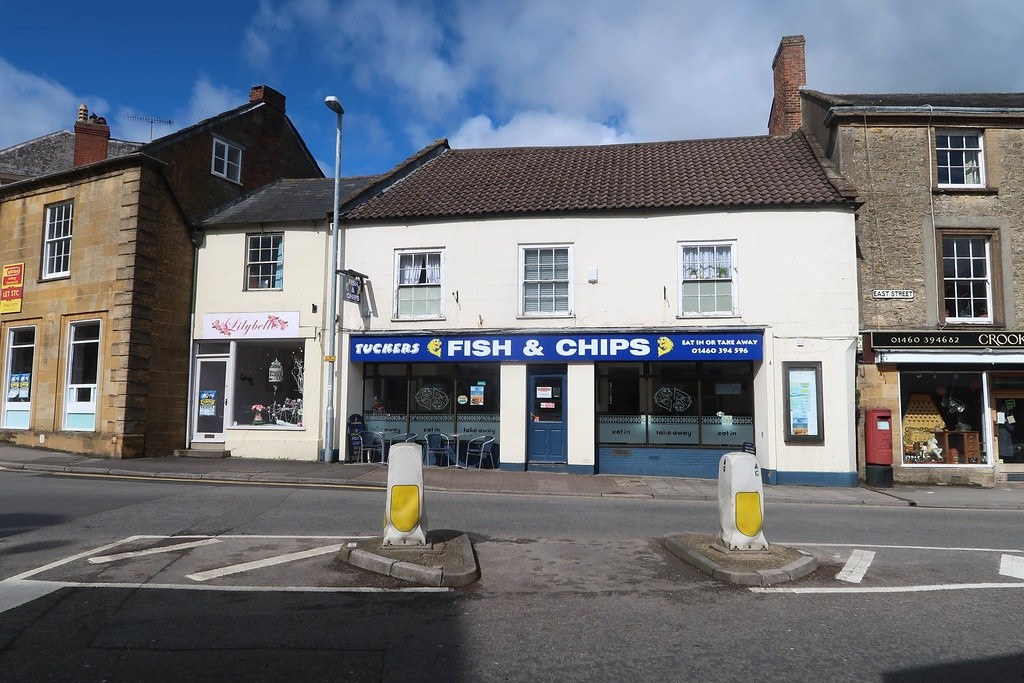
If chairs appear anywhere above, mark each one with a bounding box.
[390,432,418,445]
[466,435,496,470]
[357,431,384,463]
[424,434,450,467]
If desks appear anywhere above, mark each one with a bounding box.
[446,433,466,469]
[377,432,392,465]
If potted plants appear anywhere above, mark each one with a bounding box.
[718,268,728,278]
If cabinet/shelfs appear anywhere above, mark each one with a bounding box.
[930,431,981,464]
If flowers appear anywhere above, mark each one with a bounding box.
[252,405,265,422]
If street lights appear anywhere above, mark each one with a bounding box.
[323,94,345,462]
